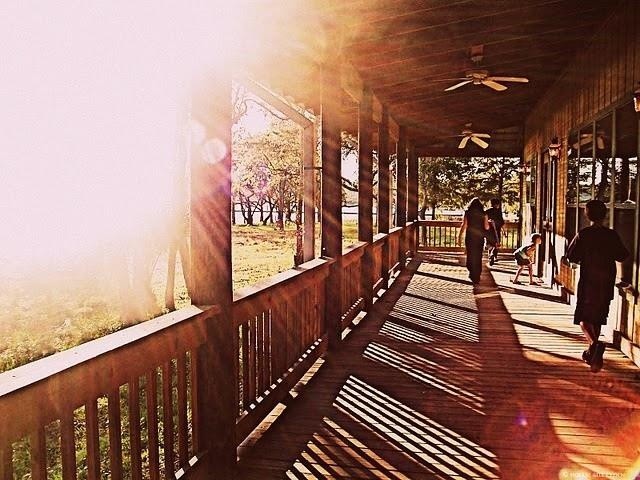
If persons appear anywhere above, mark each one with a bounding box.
[456,196,491,292]
[512,233,542,285]
[484,198,504,266]
[565,199,632,374]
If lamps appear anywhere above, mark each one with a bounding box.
[631,82,640,112]
[548,138,561,159]
[517,162,530,176]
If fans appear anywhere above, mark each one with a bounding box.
[572,125,626,150]
[434,121,492,150]
[429,55,531,94]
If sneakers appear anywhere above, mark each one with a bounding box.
[582,342,606,373]
